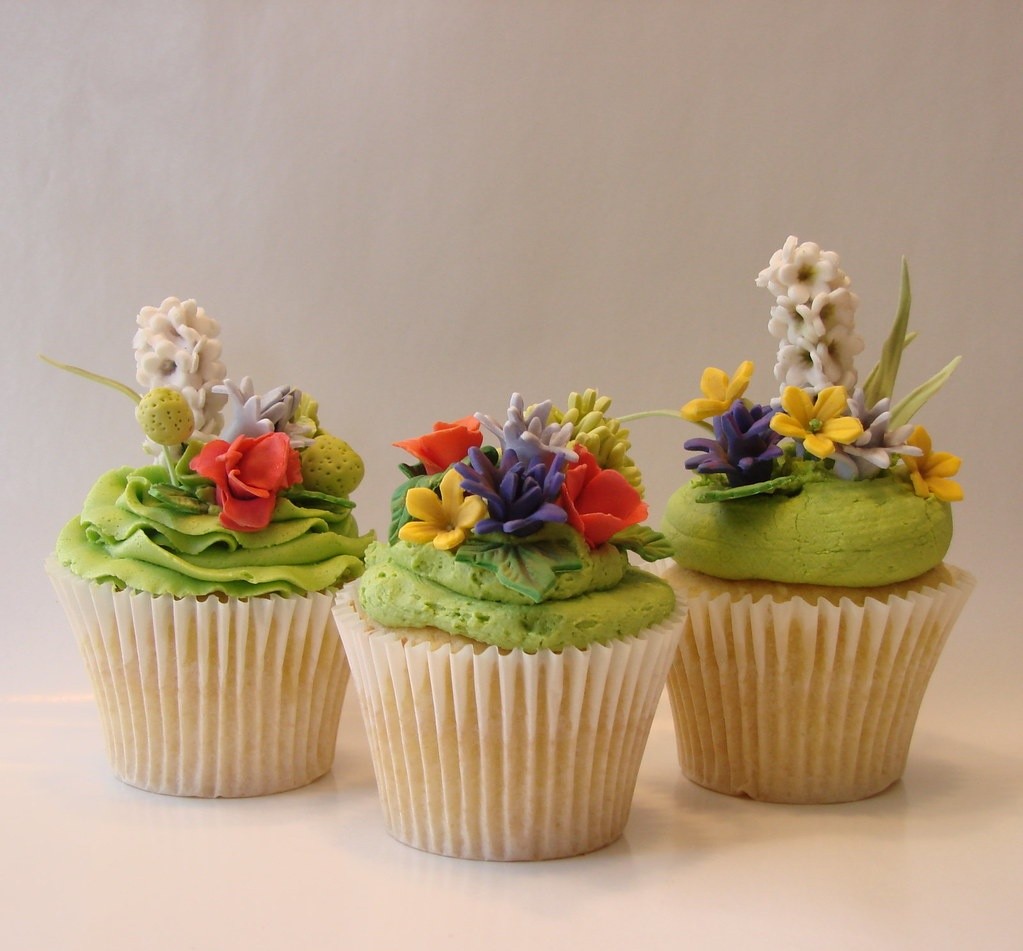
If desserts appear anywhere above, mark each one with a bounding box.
[334,387,692,862]
[34,297,378,800]
[615,235,978,806]
